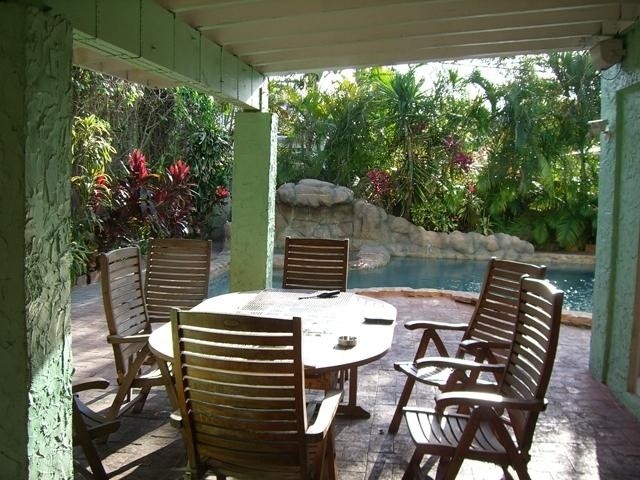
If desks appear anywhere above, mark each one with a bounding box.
[148,291,398,471]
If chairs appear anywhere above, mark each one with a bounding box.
[126,238,214,402]
[72,366,122,480]
[98,244,178,445]
[279,236,351,401]
[168,305,345,480]
[399,272,564,480]
[387,255,548,437]
